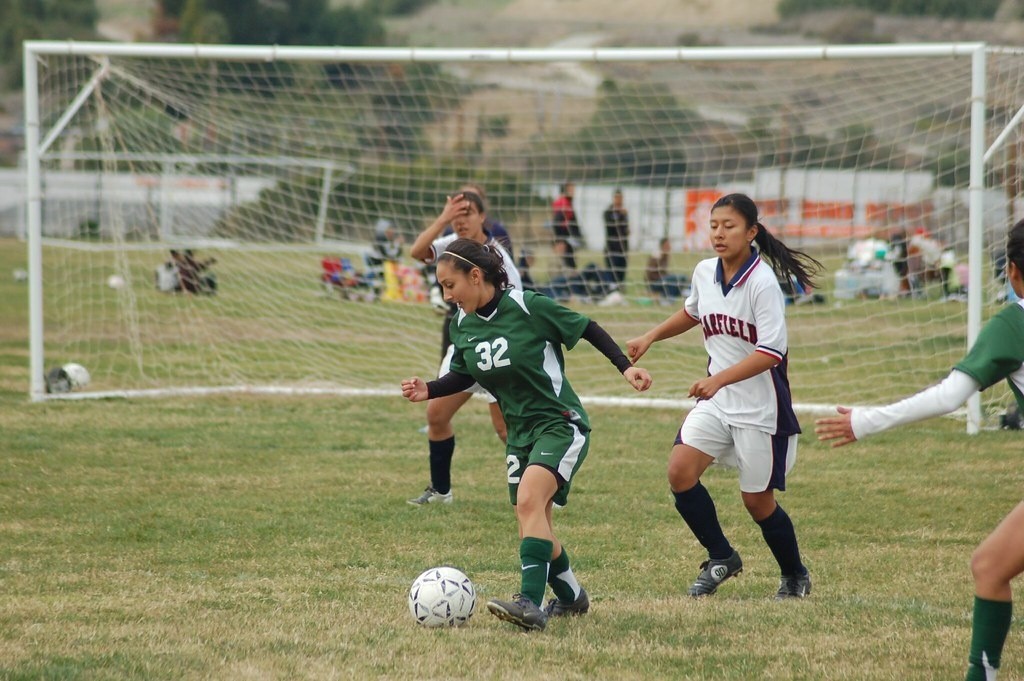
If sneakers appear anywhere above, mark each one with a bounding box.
[488,594,546,633]
[775,566,810,600]
[406,486,452,508]
[689,552,743,598]
[543,587,589,616]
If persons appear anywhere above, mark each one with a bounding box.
[408,191,525,503]
[627,191,830,600]
[399,237,654,631]
[363,180,824,315]
[155,249,217,296]
[814,218,1024,681]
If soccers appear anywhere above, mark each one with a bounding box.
[108,273,129,290]
[409,568,477,628]
[11,266,31,280]
[64,363,91,387]
